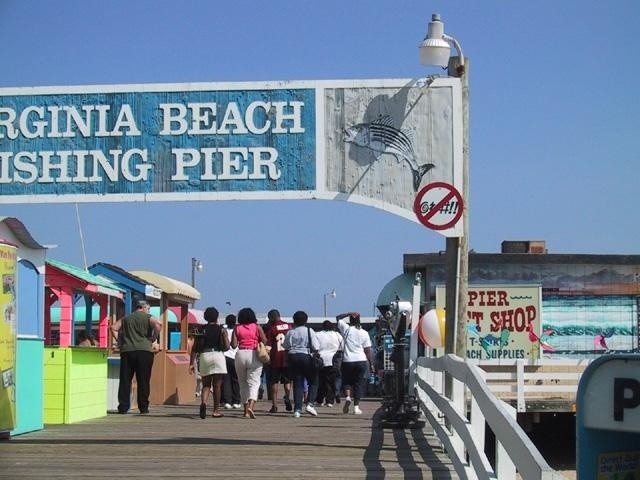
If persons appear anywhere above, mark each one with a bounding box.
[187,306,376,419]
[75,330,99,348]
[110,301,164,415]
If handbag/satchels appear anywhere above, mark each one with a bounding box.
[332,349,345,371]
[256,323,271,366]
[311,351,323,370]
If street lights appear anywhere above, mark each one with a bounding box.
[323,289,338,318]
[189,256,204,310]
[419,13,472,362]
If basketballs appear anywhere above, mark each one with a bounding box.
[418,308,446,349]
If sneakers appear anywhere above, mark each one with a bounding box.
[223,403,241,409]
[353,409,363,415]
[305,404,318,417]
[293,412,301,419]
[314,396,341,408]
[243,408,257,420]
[212,413,224,418]
[343,398,352,414]
[200,402,207,420]
[269,405,278,413]
[284,398,293,412]
